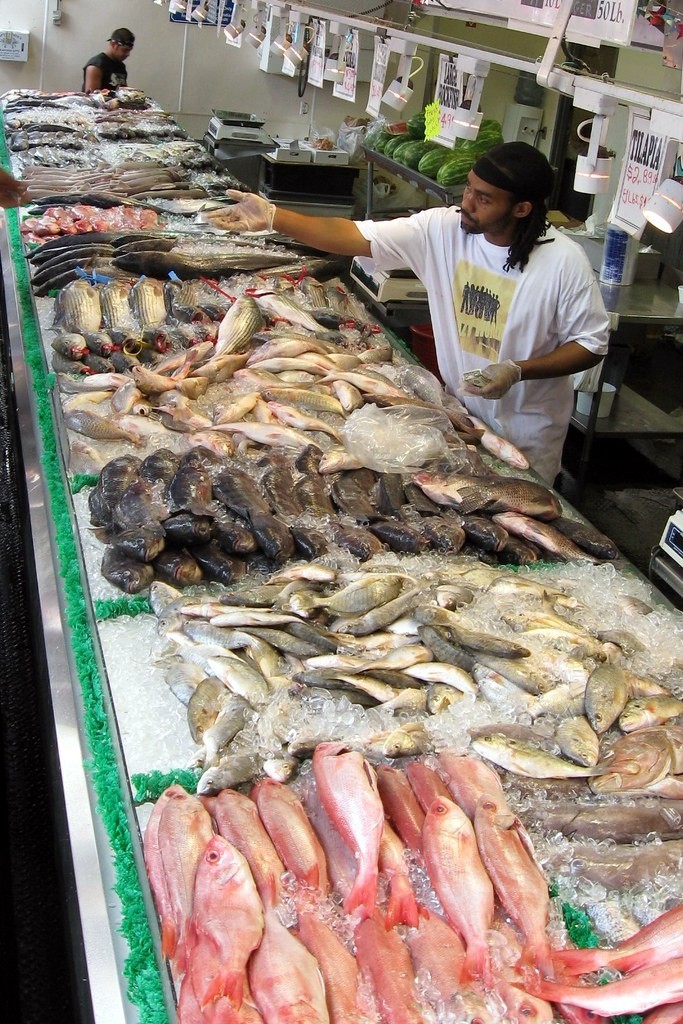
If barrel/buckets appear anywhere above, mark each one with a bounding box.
[410,321,446,387]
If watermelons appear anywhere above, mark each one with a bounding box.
[368,112,504,185]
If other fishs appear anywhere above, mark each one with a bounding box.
[0,85,683,1024]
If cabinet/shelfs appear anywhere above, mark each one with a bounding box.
[551,259,682,510]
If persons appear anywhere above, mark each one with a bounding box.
[205,141,612,488]
[82,28,135,93]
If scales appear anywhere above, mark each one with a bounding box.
[207,109,275,145]
[350,255,428,304]
[659,488,683,571]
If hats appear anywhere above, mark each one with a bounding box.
[107,28,135,48]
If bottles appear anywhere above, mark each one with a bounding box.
[598,224,640,286]
[514,70,545,107]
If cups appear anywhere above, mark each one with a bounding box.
[678,286,683,303]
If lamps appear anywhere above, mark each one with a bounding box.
[154,1,683,234]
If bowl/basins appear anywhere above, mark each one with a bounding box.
[575,381,616,418]
